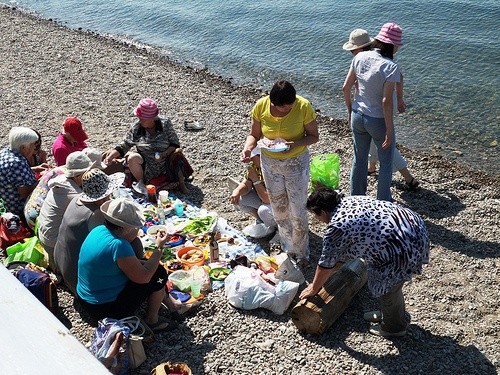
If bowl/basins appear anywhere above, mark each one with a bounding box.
[165,238,184,247]
[168,270,191,292]
[147,225,168,242]
[176,246,205,266]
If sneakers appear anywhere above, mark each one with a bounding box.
[269,231,281,244]
[364,311,382,322]
[369,324,406,336]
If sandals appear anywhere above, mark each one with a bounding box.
[401,178,418,191]
[367,168,376,176]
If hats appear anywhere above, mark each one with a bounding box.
[80,168,118,202]
[99,197,145,228]
[65,151,93,178]
[64,117,88,142]
[250,145,261,158]
[342,29,374,51]
[133,98,159,120]
[374,22,403,48]
[81,147,103,169]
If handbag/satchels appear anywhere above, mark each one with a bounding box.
[5,261,58,315]
[84,315,157,375]
[224,265,299,315]
[311,154,340,189]
[4,236,44,266]
[0,216,31,251]
[151,361,193,375]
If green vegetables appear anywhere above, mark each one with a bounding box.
[181,216,213,235]
[144,242,174,262]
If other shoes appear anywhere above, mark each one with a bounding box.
[299,259,308,267]
[142,315,169,330]
[180,183,190,194]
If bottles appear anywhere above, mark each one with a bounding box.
[147,185,157,203]
[158,206,165,225]
[175,199,183,216]
[258,259,272,272]
[209,232,219,263]
[190,273,200,299]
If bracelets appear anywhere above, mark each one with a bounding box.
[253,180,264,185]
[166,149,169,156]
[155,245,165,250]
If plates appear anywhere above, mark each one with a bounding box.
[266,142,291,153]
[145,201,232,280]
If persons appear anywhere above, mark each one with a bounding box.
[74,197,205,331]
[51,117,111,168]
[342,22,421,203]
[0,127,143,296]
[103,99,194,194]
[298,188,430,338]
[238,79,319,270]
[227,144,277,240]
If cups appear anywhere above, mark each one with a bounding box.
[159,191,168,203]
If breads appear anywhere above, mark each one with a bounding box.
[180,250,203,262]
[212,269,229,279]
